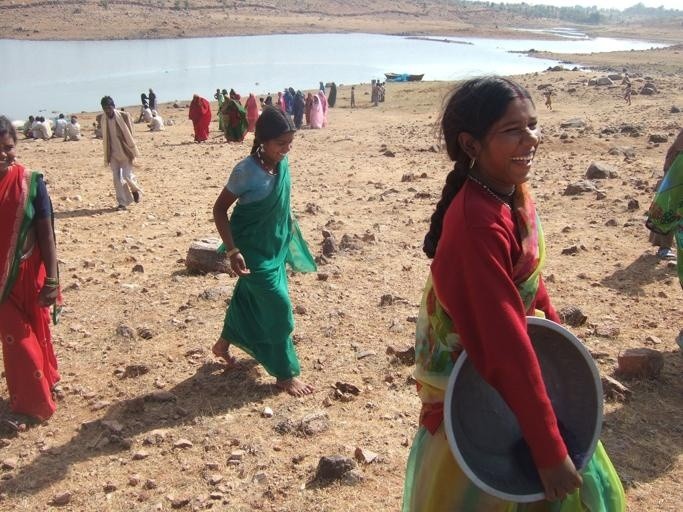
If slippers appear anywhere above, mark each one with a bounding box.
[656,248,674,257]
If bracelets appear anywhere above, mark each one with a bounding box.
[225,248,241,258]
[42,276,59,289]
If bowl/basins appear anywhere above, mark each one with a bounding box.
[441,315,604,503]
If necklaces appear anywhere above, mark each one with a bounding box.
[467,174,517,209]
[255,150,279,177]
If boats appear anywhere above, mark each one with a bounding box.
[384,72,424,82]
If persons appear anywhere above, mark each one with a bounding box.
[401,75,627,512]
[543,92,551,111]
[210,108,318,394]
[646,150,682,291]
[122,88,165,131]
[24,113,102,142]
[0,112,61,430]
[646,131,682,260]
[623,83,632,105]
[189,81,337,144]
[351,79,386,108]
[95,95,141,210]
